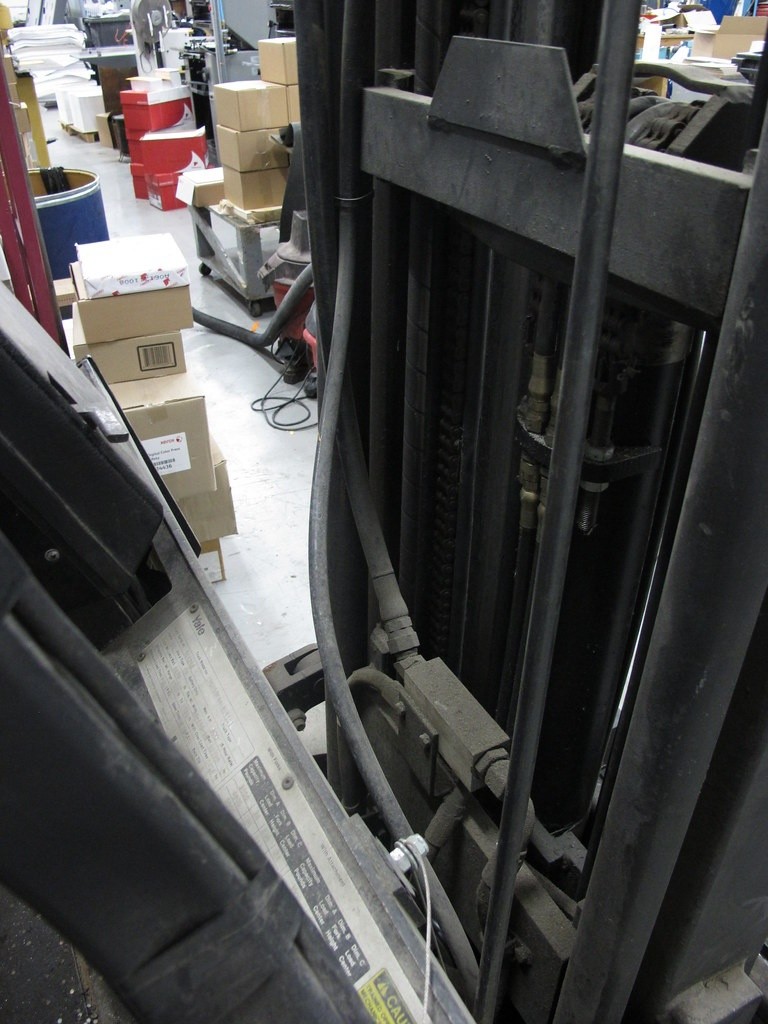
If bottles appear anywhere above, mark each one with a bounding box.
[641,21,662,62]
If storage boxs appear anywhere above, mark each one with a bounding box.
[1,54,51,170]
[120,37,301,210]
[55,84,115,148]
[69,232,239,585]
[647,3,768,61]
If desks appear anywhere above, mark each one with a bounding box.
[635,34,692,49]
